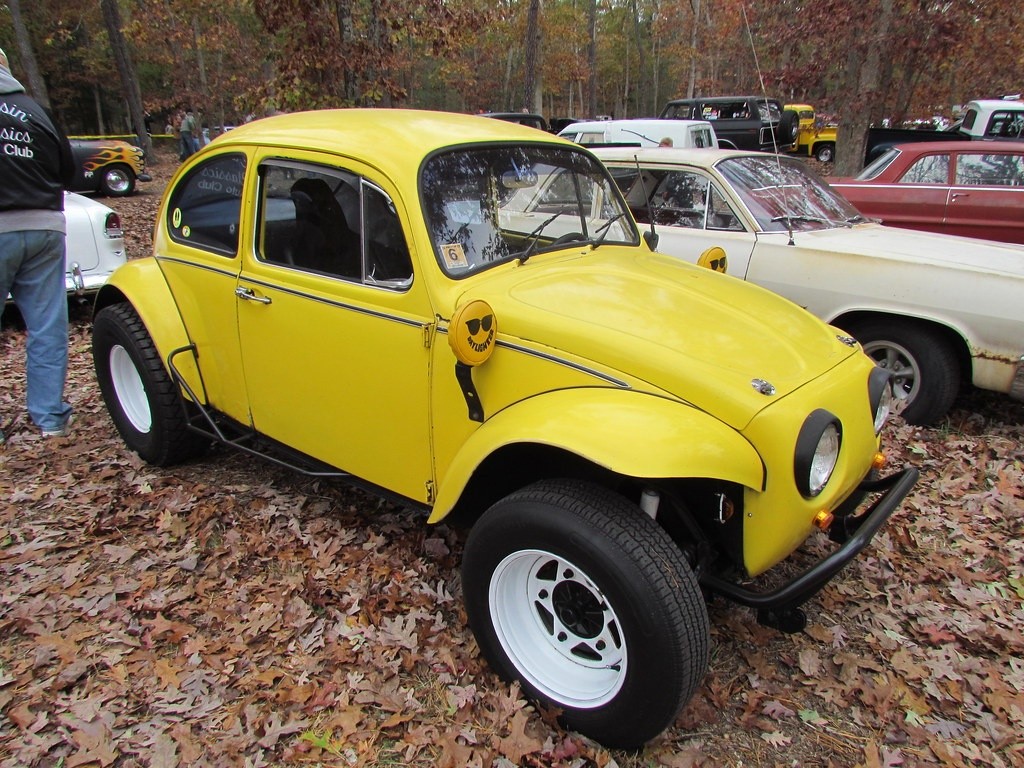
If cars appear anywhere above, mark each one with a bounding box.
[6,191,128,308]
[192,127,239,151]
[68,139,153,197]
[477,96,1024,424]
[92,109,922,748]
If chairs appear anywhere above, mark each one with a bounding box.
[422,172,509,274]
[290,176,403,284]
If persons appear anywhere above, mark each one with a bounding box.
[179,111,197,159]
[132,110,153,157]
[0,49,76,437]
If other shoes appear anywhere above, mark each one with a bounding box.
[43,415,74,438]
